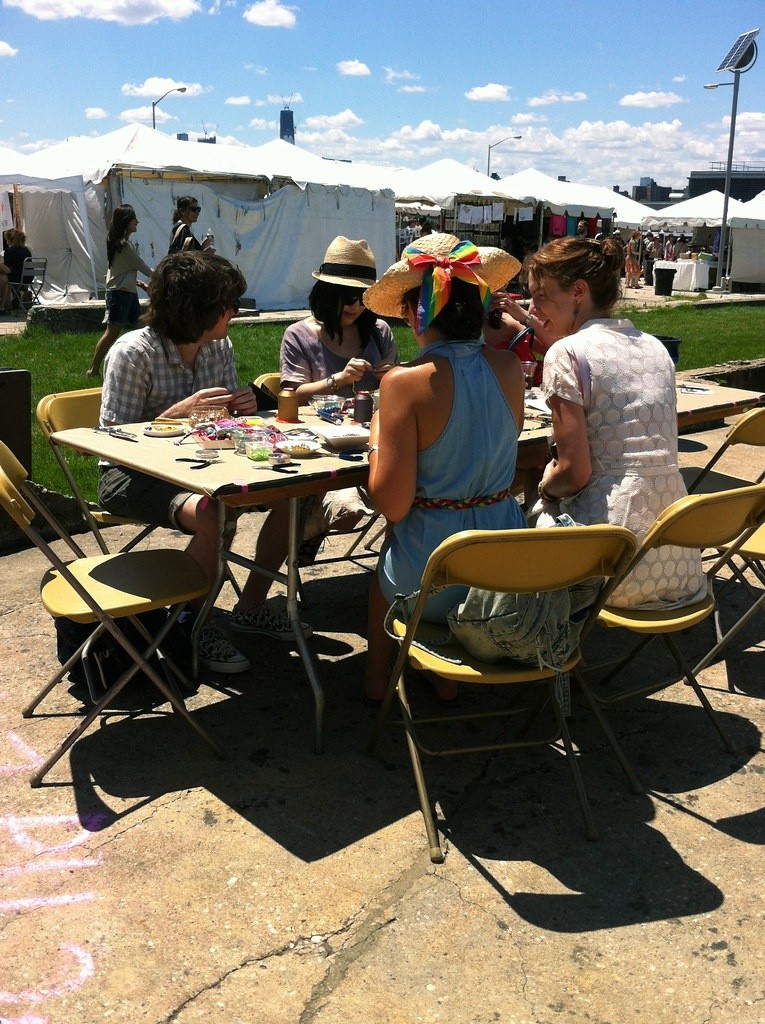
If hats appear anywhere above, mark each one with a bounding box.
[653,234,660,238]
[308,234,381,290]
[613,229,621,234]
[361,232,522,316]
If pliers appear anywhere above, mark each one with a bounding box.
[252,463,301,474]
[313,448,364,461]
[175,458,225,469]
[151,418,188,426]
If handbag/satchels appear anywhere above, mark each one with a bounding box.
[56,608,167,688]
[644,251,654,260]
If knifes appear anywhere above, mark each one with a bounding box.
[94,423,139,443]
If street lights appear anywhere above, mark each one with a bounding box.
[152,87,187,129]
[704,28,760,290]
[488,136,522,175]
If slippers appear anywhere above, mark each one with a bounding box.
[357,648,396,708]
[415,667,464,709]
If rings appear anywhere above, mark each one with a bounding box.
[508,300,512,305]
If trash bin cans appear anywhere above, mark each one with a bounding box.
[654,268,677,296]
[653,334,682,365]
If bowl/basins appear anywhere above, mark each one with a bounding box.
[309,395,345,414]
[245,440,273,461]
[231,427,271,454]
[310,424,370,450]
[190,406,229,427]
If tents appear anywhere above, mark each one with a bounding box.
[725,190,765,282]
[639,189,743,271]
[0,123,655,285]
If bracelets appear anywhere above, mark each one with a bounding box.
[524,314,533,324]
[537,481,559,503]
[367,445,378,461]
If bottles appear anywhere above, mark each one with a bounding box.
[277,387,298,422]
[206,229,215,240]
[354,390,373,423]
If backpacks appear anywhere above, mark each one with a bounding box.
[446,512,607,669]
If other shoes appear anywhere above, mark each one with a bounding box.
[285,527,330,569]
[22,291,33,310]
[0,308,11,315]
[625,284,643,289]
[86,370,100,378]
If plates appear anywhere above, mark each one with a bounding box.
[143,425,184,438]
[275,440,323,457]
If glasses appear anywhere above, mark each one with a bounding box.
[186,205,202,212]
[343,291,368,307]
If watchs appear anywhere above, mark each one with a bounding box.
[326,374,340,393]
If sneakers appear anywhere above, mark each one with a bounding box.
[229,604,313,638]
[191,620,251,674]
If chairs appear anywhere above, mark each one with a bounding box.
[8,257,48,314]
[1,372,765,864]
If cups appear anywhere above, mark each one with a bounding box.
[371,389,380,409]
[522,361,537,390]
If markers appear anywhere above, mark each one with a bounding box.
[318,411,341,424]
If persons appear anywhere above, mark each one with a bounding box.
[279,236,401,568]
[596,229,729,293]
[483,251,566,469]
[167,196,217,254]
[0,228,34,315]
[402,214,440,249]
[525,235,711,674]
[573,220,588,239]
[99,252,312,674]
[366,231,529,704]
[86,204,158,376]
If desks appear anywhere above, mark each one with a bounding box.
[52,378,765,755]
[652,258,731,291]
